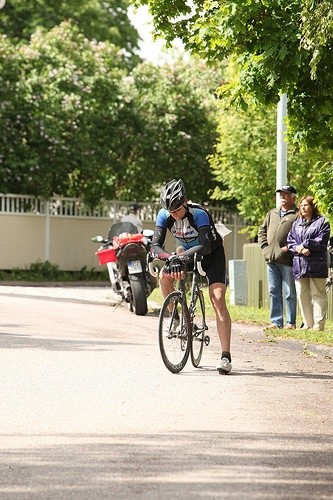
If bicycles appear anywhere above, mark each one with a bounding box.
[145,252,210,374]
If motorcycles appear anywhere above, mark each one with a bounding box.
[91,222,165,316]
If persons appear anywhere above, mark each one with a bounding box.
[287,195,331,331]
[258,186,299,331]
[150,179,232,374]
[121,204,143,234]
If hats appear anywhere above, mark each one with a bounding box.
[275,186,296,194]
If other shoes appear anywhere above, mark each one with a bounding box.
[263,325,283,331]
[283,325,296,329]
[300,325,311,331]
[310,329,324,331]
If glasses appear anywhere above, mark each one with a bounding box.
[169,207,182,213]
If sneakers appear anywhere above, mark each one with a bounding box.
[217,357,232,373]
[164,315,179,332]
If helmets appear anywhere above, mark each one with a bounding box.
[159,179,186,212]
